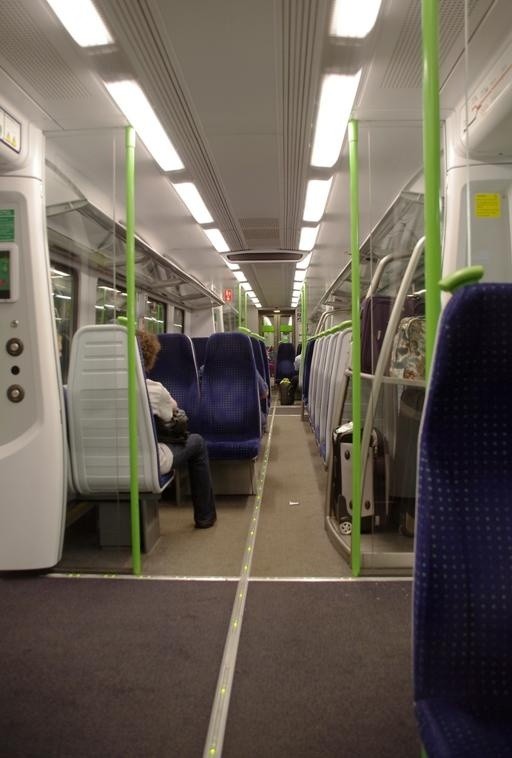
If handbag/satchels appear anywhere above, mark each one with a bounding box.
[361,294,426,380]
[153,414,188,445]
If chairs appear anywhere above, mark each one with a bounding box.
[64,284,270,553]
[275,341,296,384]
[297,283,512,757]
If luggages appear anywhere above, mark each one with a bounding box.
[279,378,294,405]
[333,420,391,535]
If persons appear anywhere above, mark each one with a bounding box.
[256,369,269,433]
[288,350,301,405]
[135,326,218,529]
